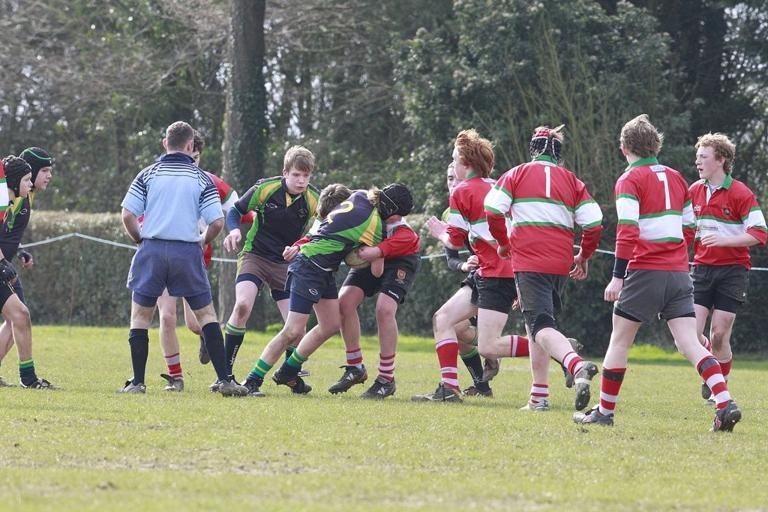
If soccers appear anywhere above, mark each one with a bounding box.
[344,248,370,270]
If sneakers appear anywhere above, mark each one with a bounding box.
[462,386,495,398]
[517,399,549,413]
[199,335,212,364]
[559,336,583,389]
[160,374,185,393]
[210,378,266,398]
[118,377,146,395]
[327,365,367,393]
[480,356,501,382]
[411,384,464,405]
[572,406,614,429]
[710,399,742,434]
[573,361,599,410]
[19,376,62,392]
[271,368,313,395]
[361,376,398,402]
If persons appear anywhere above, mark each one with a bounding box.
[413,129,584,401]
[121,121,248,395]
[484,123,603,411]
[1,154,67,391]
[573,113,741,433]
[283,182,422,396]
[683,131,768,406]
[222,145,324,389]
[441,160,500,400]
[245,182,413,396]
[1,147,53,302]
[137,129,257,391]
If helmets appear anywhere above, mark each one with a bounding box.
[19,146,52,182]
[2,155,32,196]
[381,182,413,216]
